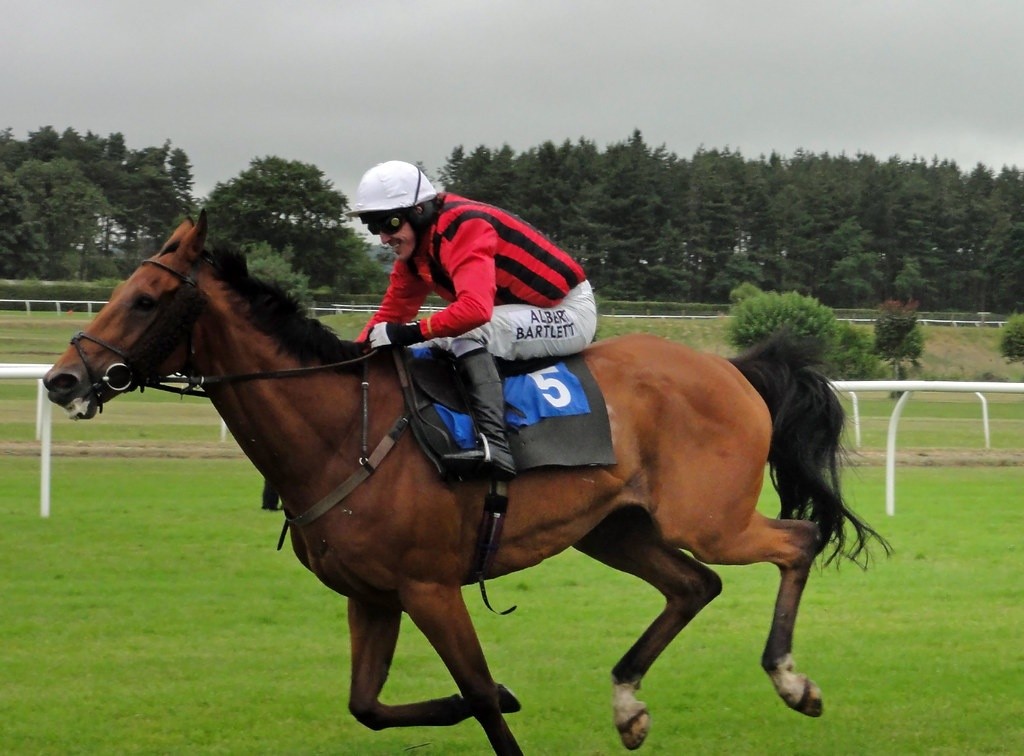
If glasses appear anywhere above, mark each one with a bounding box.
[368,213,407,235]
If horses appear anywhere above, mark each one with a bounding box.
[44,207,895,756]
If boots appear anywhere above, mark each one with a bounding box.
[441,347,517,483]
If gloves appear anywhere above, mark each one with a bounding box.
[369,319,425,352]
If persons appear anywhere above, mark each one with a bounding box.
[348,160,597,477]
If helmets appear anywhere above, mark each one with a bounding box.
[344,160,437,233]
